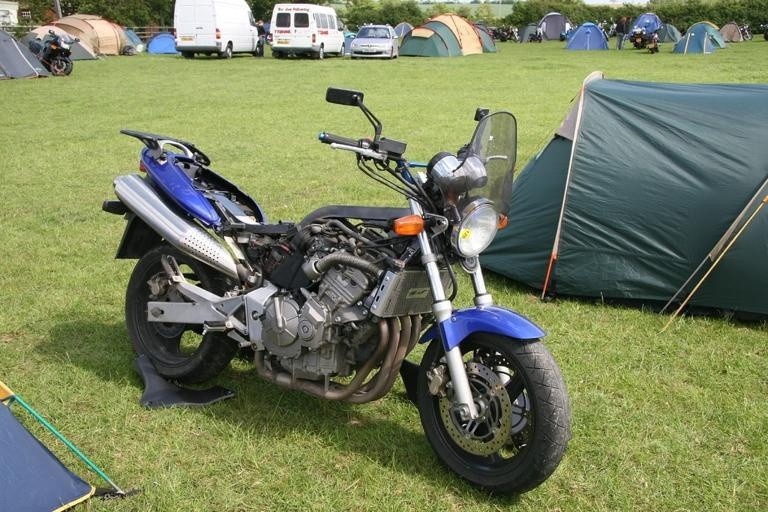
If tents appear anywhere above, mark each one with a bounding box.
[521,11,742,54]
[1,382,123,511]
[477,69,767,332]
[392,11,496,55]
[0,13,180,81]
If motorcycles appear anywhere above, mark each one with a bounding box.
[630,26,646,49]
[530,30,543,42]
[499,26,522,43]
[98,87,570,496]
[761,24,767,39]
[740,22,752,41]
[29,28,81,75]
[647,25,663,53]
[559,26,572,41]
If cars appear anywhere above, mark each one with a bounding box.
[350,25,400,61]
[475,24,495,44]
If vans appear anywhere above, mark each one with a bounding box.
[174,0,265,58]
[266,3,347,60]
